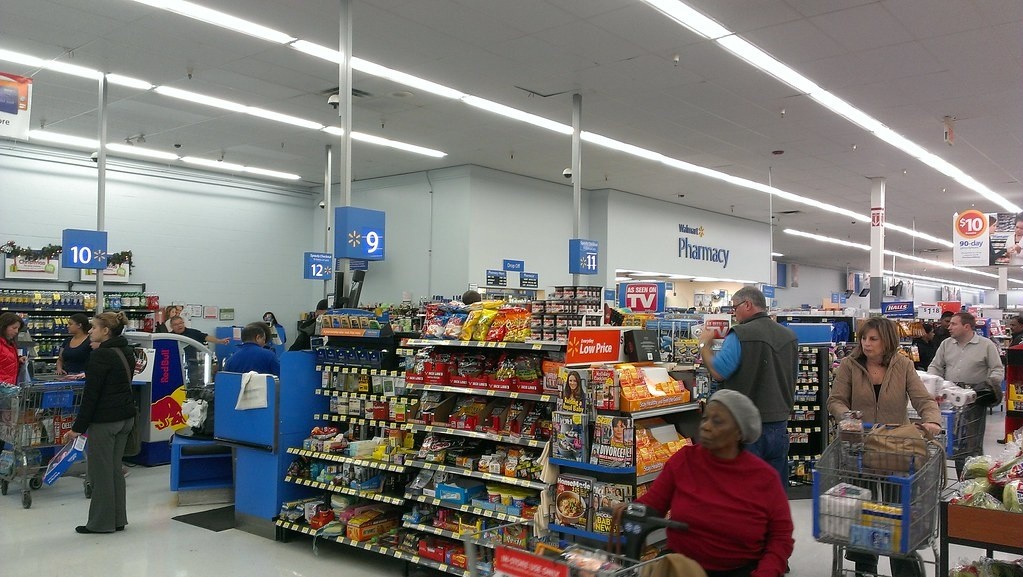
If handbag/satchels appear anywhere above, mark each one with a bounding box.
[124,406,141,456]
[974,377,1002,407]
[17,358,31,385]
[866,422,929,472]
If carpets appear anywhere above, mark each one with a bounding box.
[171,505,237,532]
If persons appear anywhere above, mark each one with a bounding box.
[605,486,609,494]
[166,305,183,333]
[615,489,623,500]
[912,323,935,372]
[826,316,942,577]
[933,310,954,353]
[601,497,610,507]
[610,417,631,447]
[246,321,275,352]
[593,496,599,512]
[989,216,1015,265]
[224,323,280,380]
[563,372,585,412]
[287,299,328,351]
[34,313,93,476]
[462,290,481,305]
[0,312,30,452]
[632,389,795,577]
[170,315,232,398]
[699,286,799,573]
[997,316,1023,444]
[263,312,286,352]
[927,312,1005,482]
[63,310,137,533]
[1005,212,1023,265]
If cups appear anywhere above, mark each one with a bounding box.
[555,491,587,525]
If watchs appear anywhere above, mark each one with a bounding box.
[700,342,707,348]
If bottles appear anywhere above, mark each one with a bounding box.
[0,289,159,358]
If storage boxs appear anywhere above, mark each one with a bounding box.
[480,521,503,544]
[630,418,686,476]
[503,527,527,550]
[407,363,575,442]
[5,250,59,280]
[80,253,129,283]
[439,479,485,505]
[624,329,662,362]
[620,375,690,412]
[347,520,399,542]
[419,539,452,562]
[459,517,481,538]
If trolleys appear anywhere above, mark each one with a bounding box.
[462,504,689,577]
[0,374,93,509]
[810,423,946,577]
[906,383,989,491]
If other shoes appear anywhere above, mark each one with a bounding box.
[76,526,114,533]
[997,439,1005,444]
[116,525,124,531]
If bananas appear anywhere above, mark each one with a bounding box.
[947,559,1023,577]
[953,456,1023,513]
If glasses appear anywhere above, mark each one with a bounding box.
[731,300,745,312]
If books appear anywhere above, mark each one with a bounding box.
[551,368,633,537]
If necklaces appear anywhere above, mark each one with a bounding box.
[870,363,883,374]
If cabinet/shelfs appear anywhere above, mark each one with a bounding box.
[0,278,155,359]
[271,321,707,577]
[656,307,1023,577]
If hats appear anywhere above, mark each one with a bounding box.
[708,388,762,445]
[317,299,328,310]
